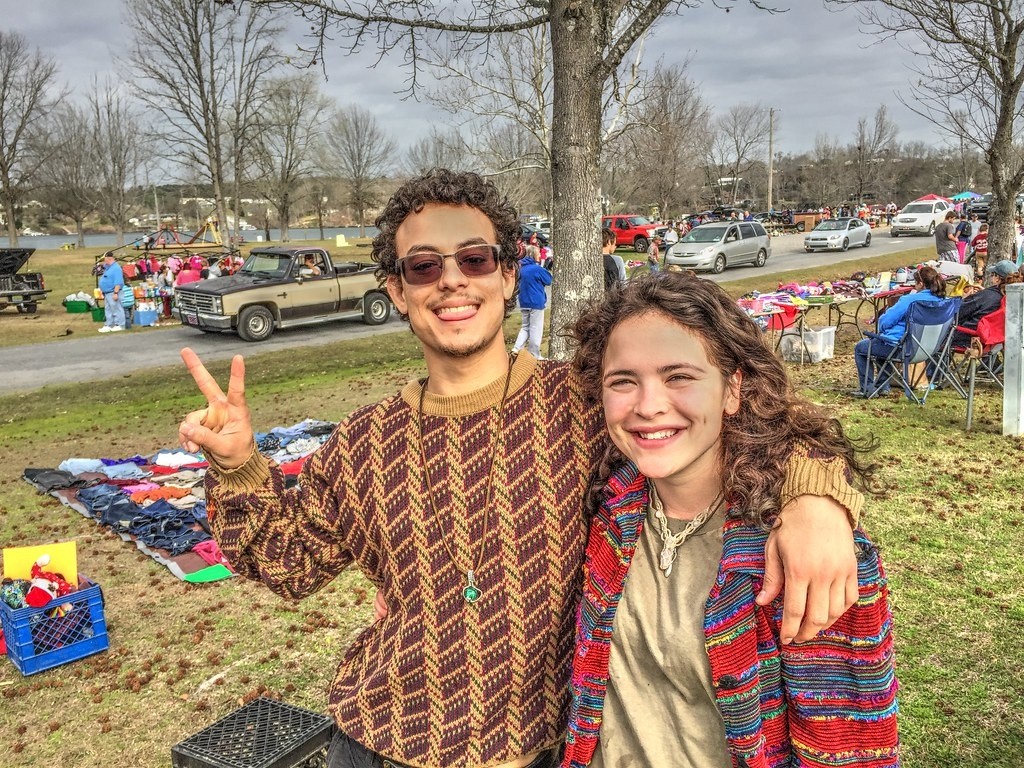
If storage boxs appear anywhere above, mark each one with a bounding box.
[777,326,837,364]
[807,296,834,304]
[0,572,109,677]
[63,300,90,313]
[892,361,931,387]
[133,310,158,326]
[90,307,106,322]
[737,299,764,314]
[324,263,358,274]
[171,696,338,768]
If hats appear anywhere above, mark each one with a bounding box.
[653,235,662,240]
[989,260,1019,277]
[105,252,113,257]
[971,213,977,217]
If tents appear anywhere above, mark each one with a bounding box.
[913,191,981,204]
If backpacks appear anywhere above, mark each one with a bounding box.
[961,221,971,236]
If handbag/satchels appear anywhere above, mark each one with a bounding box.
[647,247,658,265]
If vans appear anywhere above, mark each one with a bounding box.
[664,220,773,274]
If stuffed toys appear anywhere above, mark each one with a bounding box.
[25,554,71,607]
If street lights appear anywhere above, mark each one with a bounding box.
[768,109,781,219]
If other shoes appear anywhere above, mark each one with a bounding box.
[850,390,878,398]
[880,384,892,395]
[537,357,547,361]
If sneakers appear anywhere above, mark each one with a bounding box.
[97,325,111,333]
[111,325,125,331]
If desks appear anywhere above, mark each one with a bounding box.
[131,295,175,324]
[95,299,104,307]
[750,276,962,366]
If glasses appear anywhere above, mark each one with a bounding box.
[394,239,501,287]
[306,257,314,260]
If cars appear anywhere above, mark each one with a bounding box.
[967,193,1024,225]
[891,199,953,237]
[527,222,550,234]
[602,215,668,253]
[804,217,871,253]
[521,223,549,247]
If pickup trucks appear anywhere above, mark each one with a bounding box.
[170,246,393,342]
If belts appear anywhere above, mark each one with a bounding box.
[103,288,122,294]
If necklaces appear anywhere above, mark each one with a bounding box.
[649,481,726,578]
[418,353,513,603]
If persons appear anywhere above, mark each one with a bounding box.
[850,267,946,399]
[602,226,627,291]
[560,265,901,768]
[935,199,1024,280]
[98,252,134,332]
[807,200,897,226]
[648,211,753,267]
[927,259,1024,384]
[299,254,321,276]
[510,232,554,361]
[135,233,166,250]
[157,261,239,319]
[179,168,866,768]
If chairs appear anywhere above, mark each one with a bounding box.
[862,295,1008,431]
